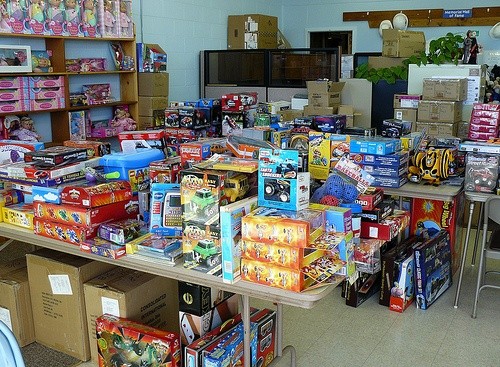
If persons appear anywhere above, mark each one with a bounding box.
[70,117,82,137]
[31,56,51,71]
[64,0,80,26]
[29,0,45,23]
[464,29,472,64]
[81,59,104,71]
[0,51,28,66]
[120,3,130,34]
[7,0,25,23]
[113,111,139,131]
[86,86,108,99]
[11,116,43,143]
[47,0,63,25]
[83,0,96,27]
[104,0,117,33]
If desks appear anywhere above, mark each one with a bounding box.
[453,191,497,309]
[383,183,462,202]
[0,222,341,367]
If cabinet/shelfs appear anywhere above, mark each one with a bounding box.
[0,23,138,149]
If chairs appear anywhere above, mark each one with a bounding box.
[472,196,500,319]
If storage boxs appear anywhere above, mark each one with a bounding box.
[0,0,500,367]
